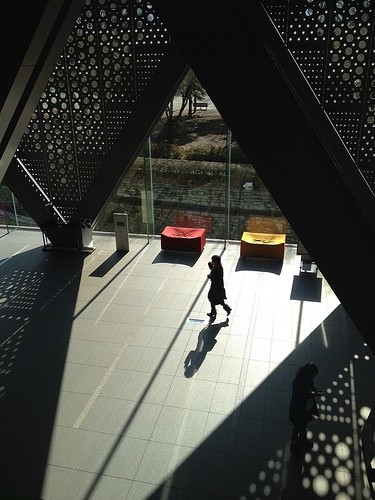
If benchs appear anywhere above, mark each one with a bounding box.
[160,225,207,253]
[241,232,286,259]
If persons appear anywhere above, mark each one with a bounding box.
[207,255,233,318]
[289,363,321,460]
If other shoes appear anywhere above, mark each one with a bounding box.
[207,313,215,317]
[226,308,232,316]
